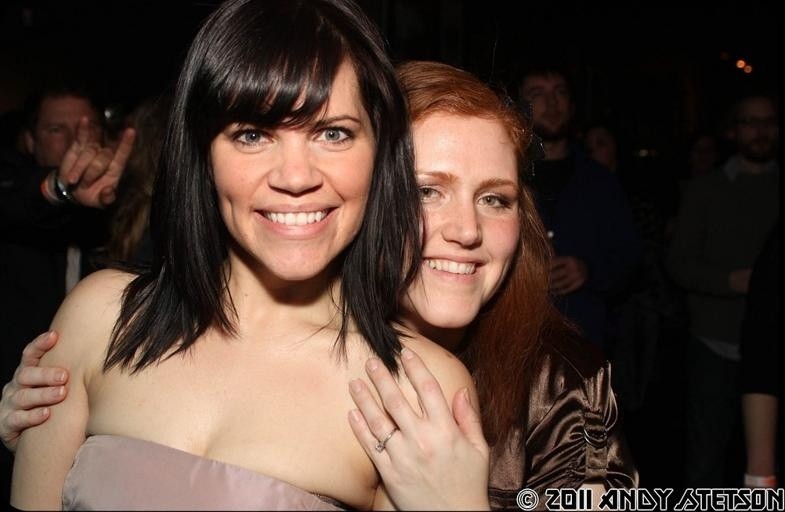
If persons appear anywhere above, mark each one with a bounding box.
[2,2,783,512]
